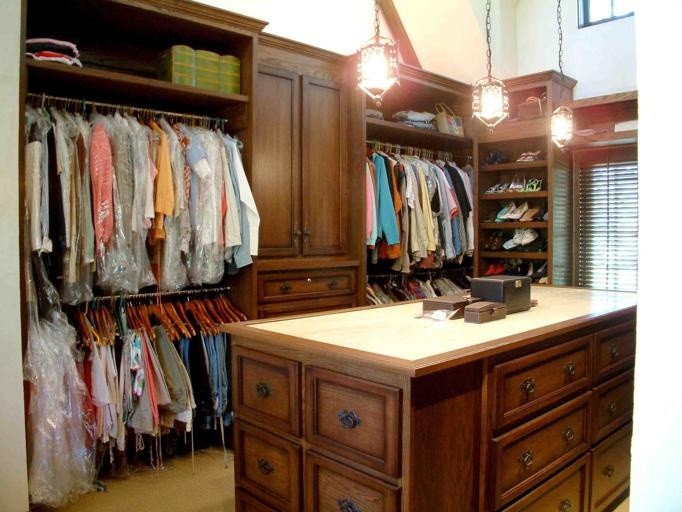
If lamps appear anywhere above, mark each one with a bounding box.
[471,0,509,131]
[359,1,400,107]
[550,1,574,150]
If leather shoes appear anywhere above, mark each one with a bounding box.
[481,149,548,284]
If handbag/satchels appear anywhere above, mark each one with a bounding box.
[434,101,465,136]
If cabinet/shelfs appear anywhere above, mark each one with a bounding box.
[254,33,359,267]
[358,52,478,306]
[478,69,577,287]
[219,283,636,512]
[238,264,367,321]
[22,1,268,510]
